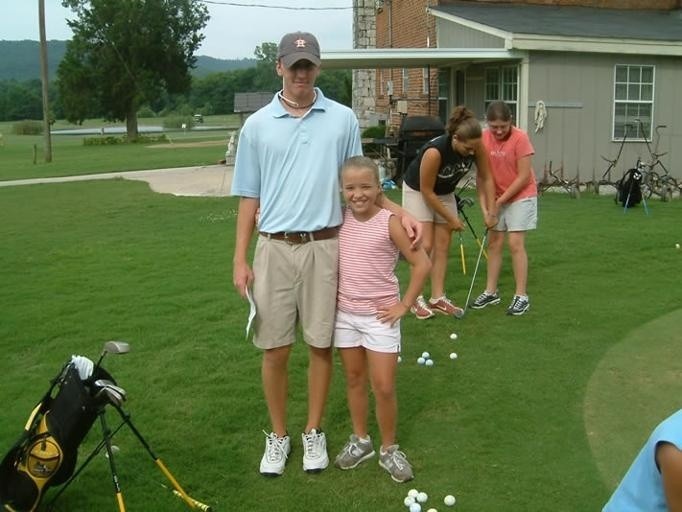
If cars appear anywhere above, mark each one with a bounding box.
[194,114,203,123]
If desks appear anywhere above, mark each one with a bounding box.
[362,137,386,157]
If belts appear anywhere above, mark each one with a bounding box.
[260,227,338,244]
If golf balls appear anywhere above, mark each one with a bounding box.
[450,353,457,360]
[397,356,401,362]
[676,244,680,249]
[404,489,456,512]
[451,334,457,339]
[417,352,434,366]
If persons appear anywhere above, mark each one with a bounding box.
[334,155,433,484]
[469,99,539,316]
[402,106,500,320]
[599,409,682,512]
[231,31,425,477]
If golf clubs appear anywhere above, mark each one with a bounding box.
[72,342,210,510]
[454,227,488,318]
[623,158,639,214]
[456,177,488,274]
[637,161,649,215]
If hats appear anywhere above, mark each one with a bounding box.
[278,32,320,70]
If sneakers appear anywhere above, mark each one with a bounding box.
[378,444,415,483]
[334,434,375,470]
[506,293,530,315]
[259,429,292,476]
[469,291,501,309]
[410,294,462,319]
[301,427,330,474]
